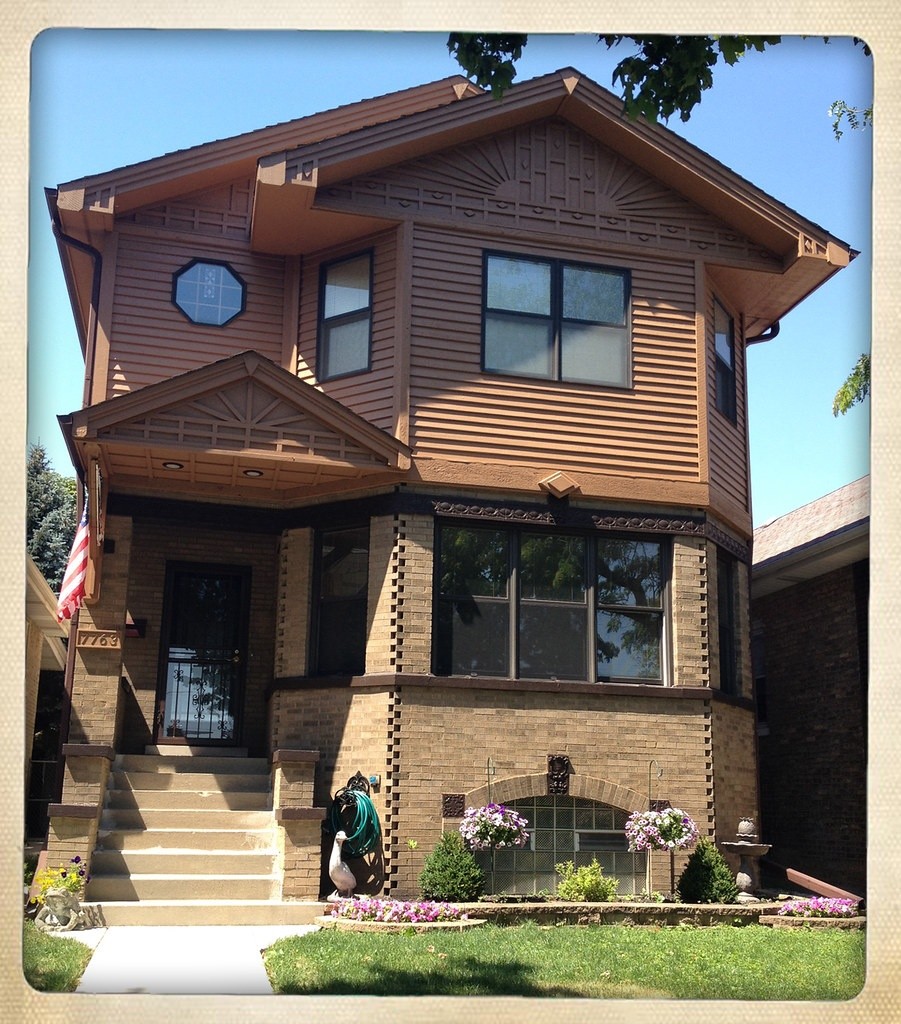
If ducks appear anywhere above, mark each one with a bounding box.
[329,831,356,898]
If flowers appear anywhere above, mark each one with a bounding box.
[625,808,700,852]
[459,807,528,849]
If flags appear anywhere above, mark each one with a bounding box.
[56,489,89,623]
